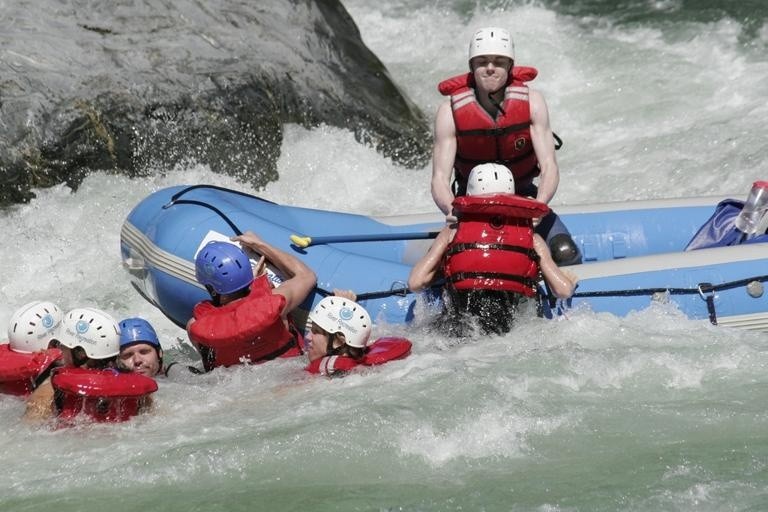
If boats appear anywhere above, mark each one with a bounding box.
[121,184,768,349]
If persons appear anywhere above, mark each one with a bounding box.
[406,162,576,341]
[304,296,415,378]
[428,25,584,268]
[184,224,320,369]
[22,305,155,428]
[0,299,67,398]
[115,315,190,384]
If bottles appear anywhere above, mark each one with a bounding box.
[735,181,768,235]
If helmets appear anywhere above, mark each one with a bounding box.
[54,307,121,360]
[8,300,63,354]
[465,162,516,198]
[195,241,254,295]
[467,26,517,67]
[310,295,373,349]
[118,318,160,349]
